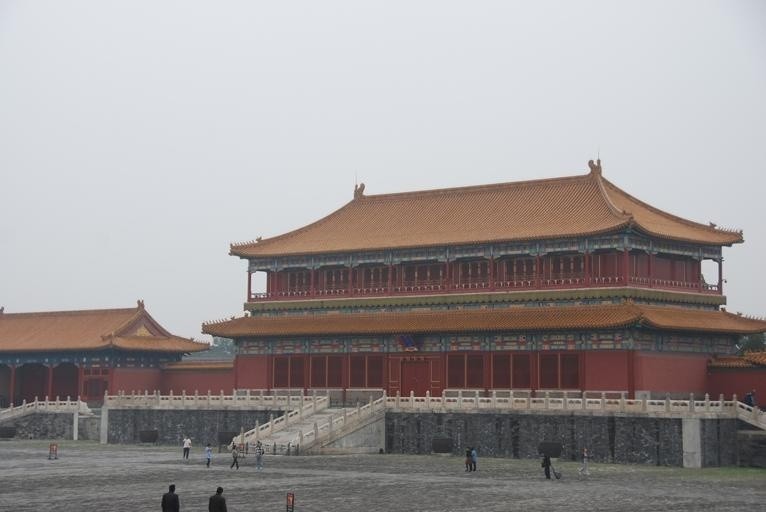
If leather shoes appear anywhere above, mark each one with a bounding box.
[230,465,240,469]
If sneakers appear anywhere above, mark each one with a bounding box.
[256,466,264,470]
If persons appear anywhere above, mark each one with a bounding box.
[182,434,193,461]
[255,440,265,470]
[206,443,213,469]
[464,446,473,473]
[229,445,241,471]
[541,452,555,478]
[207,486,228,511]
[161,484,181,512]
[470,447,477,472]
[578,446,591,475]
[744,389,757,411]
